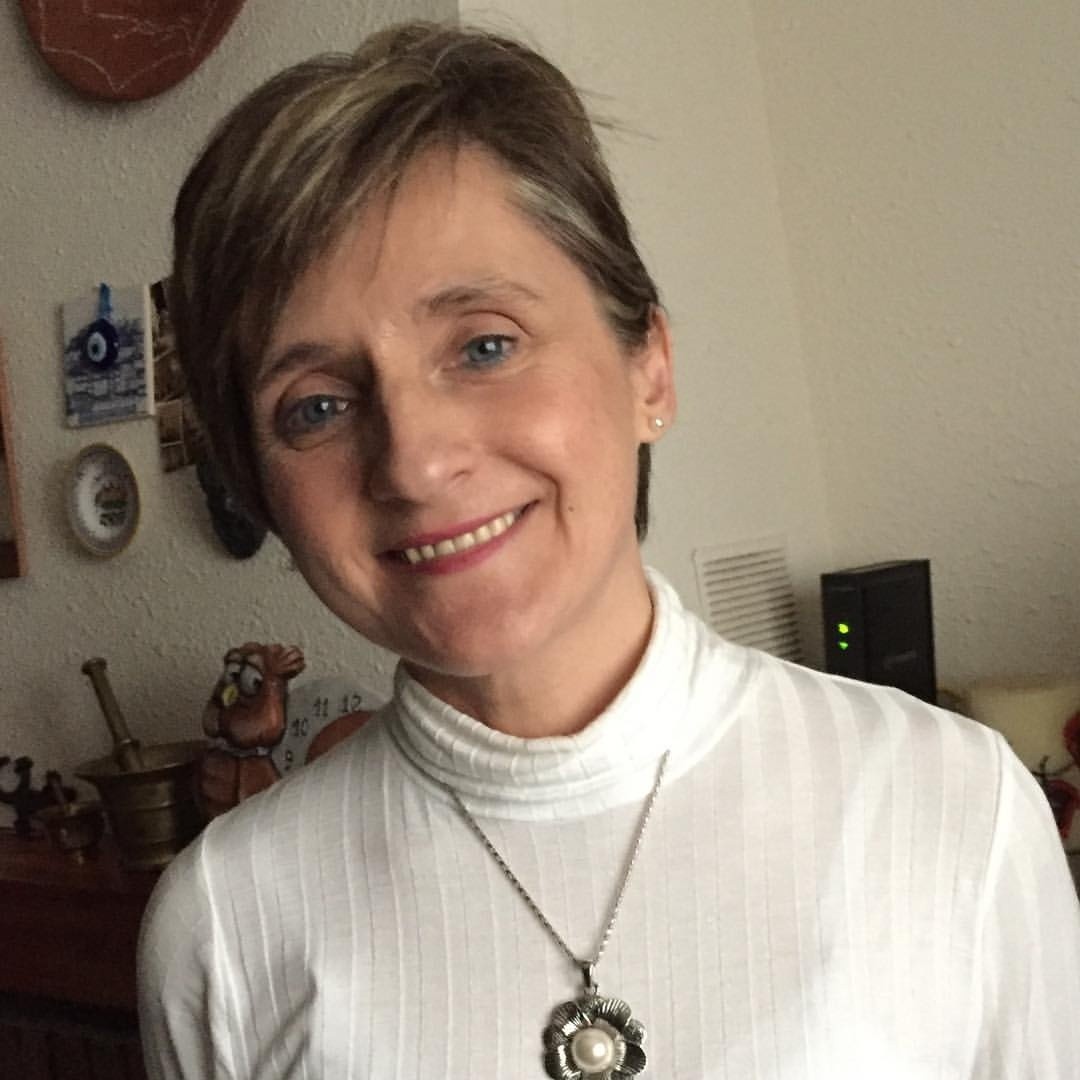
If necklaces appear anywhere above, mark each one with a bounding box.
[441,745,680,1080]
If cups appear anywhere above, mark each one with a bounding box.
[77,737,207,877]
[37,801,103,869]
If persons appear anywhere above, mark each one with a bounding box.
[126,13,1078,1080]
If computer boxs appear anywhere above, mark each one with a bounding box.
[822,557,937,706]
